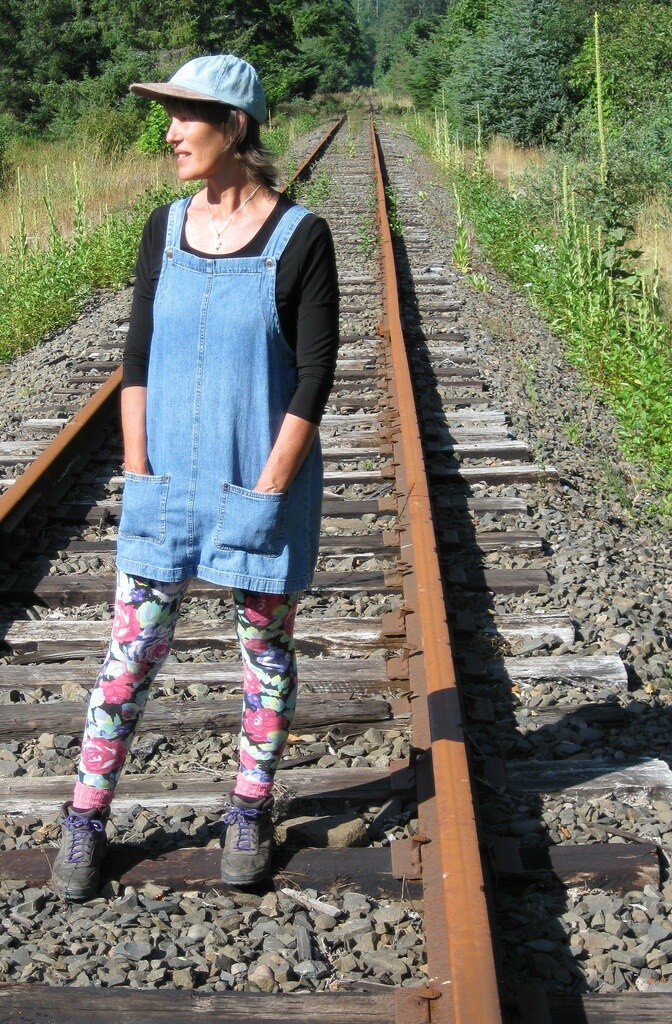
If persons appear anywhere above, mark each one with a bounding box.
[53,52,329,900]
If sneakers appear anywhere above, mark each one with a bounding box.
[53,800,111,898]
[219,791,275,884]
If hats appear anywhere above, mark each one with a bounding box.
[129,55,268,124]
